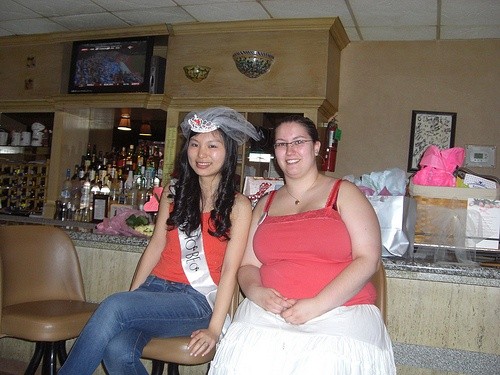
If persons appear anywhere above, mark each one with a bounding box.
[206,115,397,375]
[55,106,257,375]
[75,48,125,87]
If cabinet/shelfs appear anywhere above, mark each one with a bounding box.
[0,17,350,236]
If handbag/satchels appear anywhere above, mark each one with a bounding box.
[97,207,155,239]
[366,195,410,257]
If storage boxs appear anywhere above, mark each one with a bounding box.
[408,178,500,252]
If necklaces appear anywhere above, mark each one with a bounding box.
[286,172,320,204]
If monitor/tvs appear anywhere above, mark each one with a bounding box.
[68,35,155,93]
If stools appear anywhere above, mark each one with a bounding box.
[141,282,239,375]
[0,226,102,375]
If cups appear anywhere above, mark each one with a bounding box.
[0,130,43,146]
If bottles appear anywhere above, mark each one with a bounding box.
[53,144,164,226]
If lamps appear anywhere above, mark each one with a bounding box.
[183,65,211,83]
[117,117,132,131]
[233,50,275,78]
[139,120,151,136]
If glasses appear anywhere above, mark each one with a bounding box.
[274,139,314,149]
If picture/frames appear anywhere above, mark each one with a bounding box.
[407,110,457,173]
